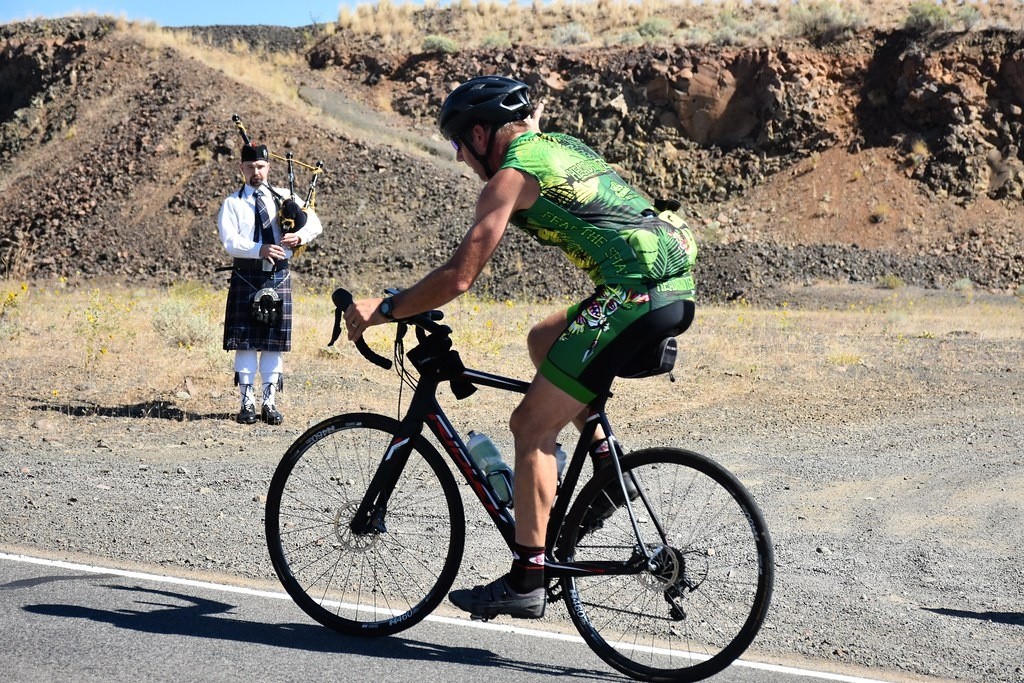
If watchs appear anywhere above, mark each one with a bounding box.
[379,297,397,324]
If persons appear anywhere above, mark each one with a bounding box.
[343,75,695,617]
[219,144,323,425]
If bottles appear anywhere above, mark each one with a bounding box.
[555,443,567,479]
[466,430,515,501]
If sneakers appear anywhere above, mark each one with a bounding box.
[581,473,639,529]
[449,573,547,618]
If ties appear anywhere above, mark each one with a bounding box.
[252,189,275,245]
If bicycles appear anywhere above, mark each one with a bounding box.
[263,287,775,683]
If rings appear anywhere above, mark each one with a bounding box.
[351,323,357,328]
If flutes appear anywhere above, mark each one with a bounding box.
[232,113,324,281]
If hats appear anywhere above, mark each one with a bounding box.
[241,144,269,163]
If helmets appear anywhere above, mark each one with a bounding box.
[437,75,533,140]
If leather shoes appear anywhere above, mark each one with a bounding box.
[239,404,257,423]
[261,405,282,424]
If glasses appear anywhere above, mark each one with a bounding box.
[451,133,464,149]
